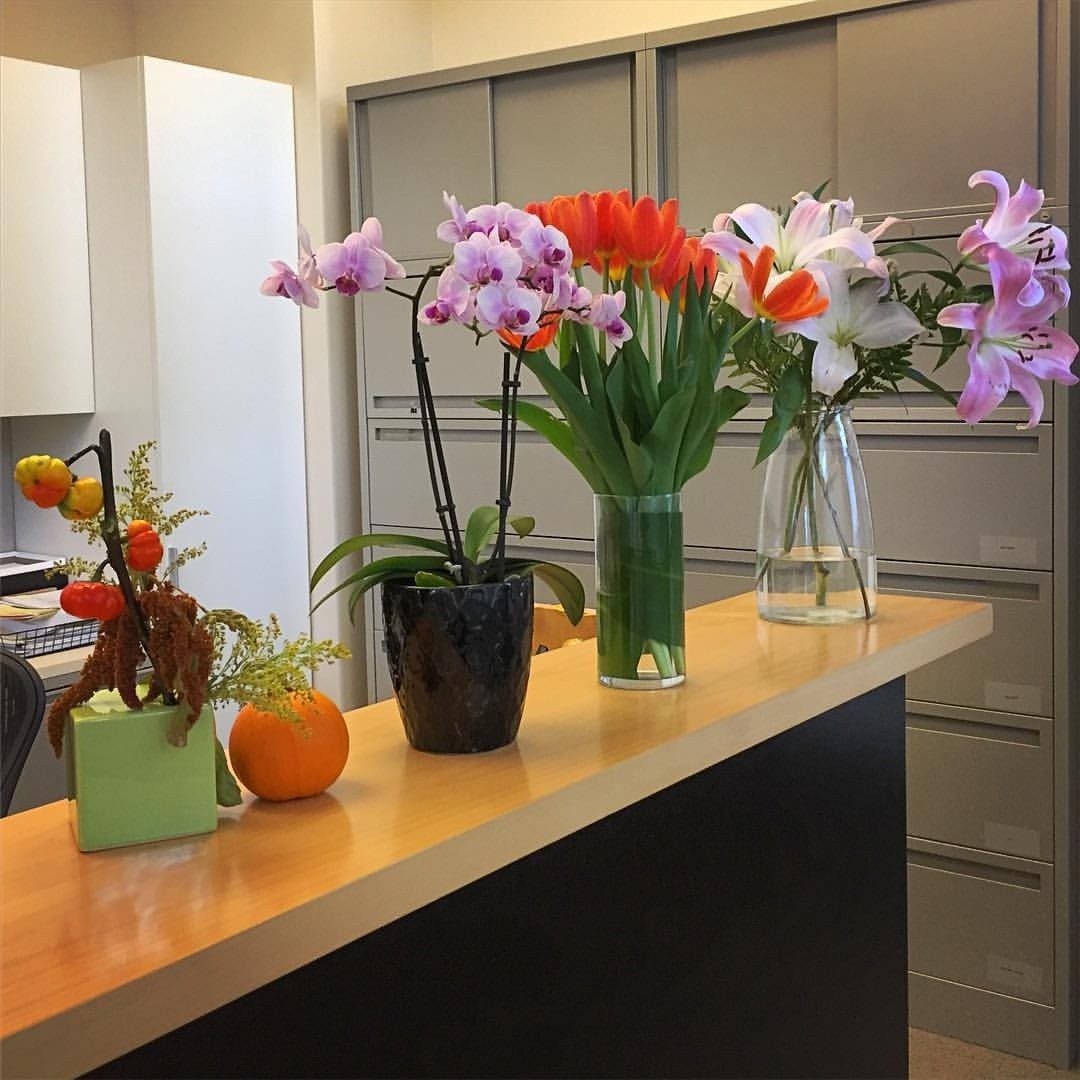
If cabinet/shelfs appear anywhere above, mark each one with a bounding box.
[347,0,1080,1072]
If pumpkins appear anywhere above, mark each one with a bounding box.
[60,559,124,619]
[229,689,350,800]
[14,455,102,520]
[120,521,163,568]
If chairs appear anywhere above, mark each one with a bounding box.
[0,645,46,817]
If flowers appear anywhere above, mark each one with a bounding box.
[700,168,1079,470]
[260,190,631,627]
[481,195,803,489]
[16,424,348,758]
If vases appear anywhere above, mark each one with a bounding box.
[756,399,878,625]
[592,490,687,689]
[63,687,218,853]
[376,573,534,753]
